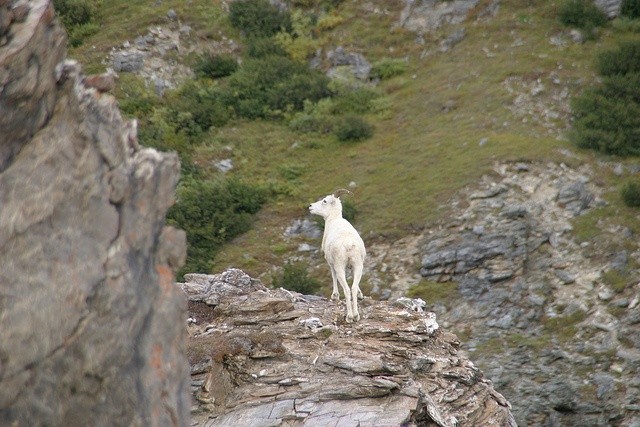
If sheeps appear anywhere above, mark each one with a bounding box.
[308,187,367,323]
